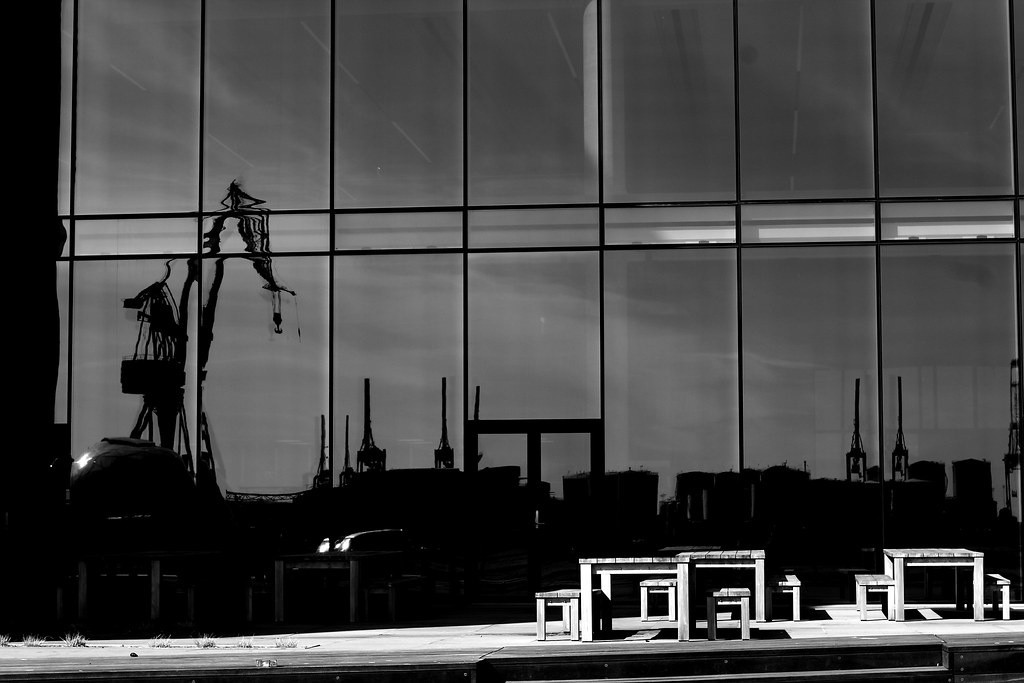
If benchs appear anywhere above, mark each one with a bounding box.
[855,574,896,621]
[707,587,750,641]
[984,573,1012,620]
[764,570,802,621]
[640,579,678,622]
[534,589,602,640]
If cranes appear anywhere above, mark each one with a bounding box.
[118,177,284,484]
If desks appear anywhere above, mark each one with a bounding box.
[676,550,766,624]
[658,546,720,556]
[276,550,401,624]
[48,551,220,635]
[882,548,985,621]
[578,558,691,641]
